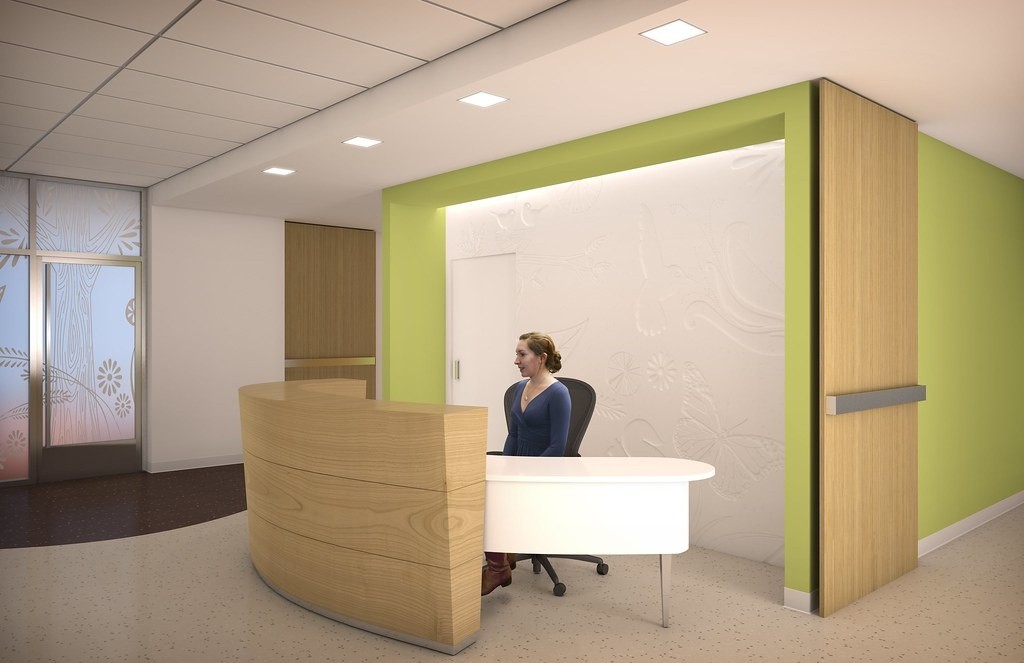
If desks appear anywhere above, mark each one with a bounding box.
[486,454,715,629]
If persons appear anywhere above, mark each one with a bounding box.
[481,332,572,596]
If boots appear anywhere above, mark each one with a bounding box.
[481,552,516,596]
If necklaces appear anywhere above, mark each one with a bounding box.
[524,386,535,400]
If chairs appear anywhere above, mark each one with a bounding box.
[486,377,609,596]
